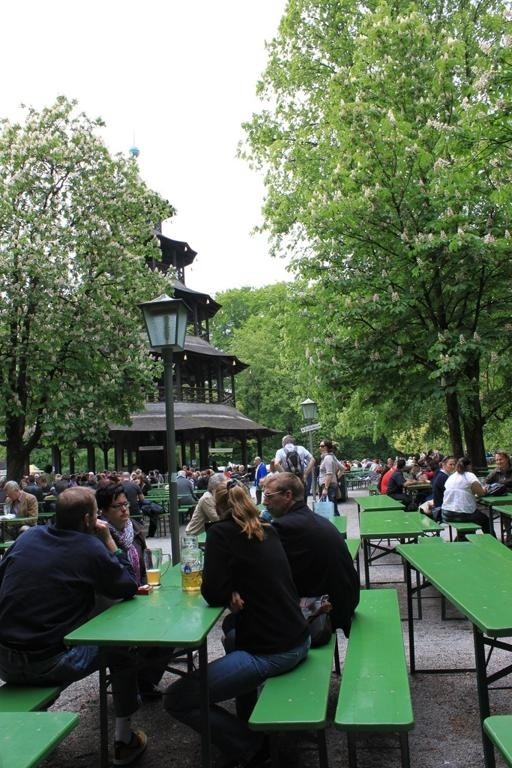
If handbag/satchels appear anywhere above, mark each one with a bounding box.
[313,501,335,520]
[487,482,506,496]
[431,507,441,521]
[299,595,332,649]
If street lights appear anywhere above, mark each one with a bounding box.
[136,292,192,565]
[300,397,318,501]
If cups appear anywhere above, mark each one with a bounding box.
[180,535,199,549]
[180,548,204,593]
[3,505,11,516]
[143,548,171,589]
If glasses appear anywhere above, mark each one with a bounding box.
[319,445,326,448]
[112,501,130,512]
[262,491,281,500]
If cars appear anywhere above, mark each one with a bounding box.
[485,452,496,464]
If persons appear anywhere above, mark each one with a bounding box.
[0,450,512,537]
[95,482,148,586]
[259,471,360,632]
[319,440,345,516]
[0,487,148,765]
[165,478,311,768]
[274,434,315,506]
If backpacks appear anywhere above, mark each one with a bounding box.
[283,446,304,479]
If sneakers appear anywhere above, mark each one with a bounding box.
[113,730,147,765]
